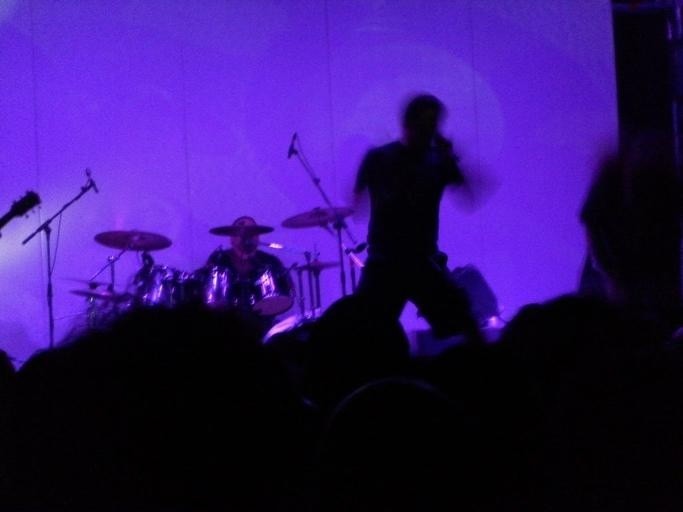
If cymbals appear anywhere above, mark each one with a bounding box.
[209,224,274,237]
[297,260,342,270]
[71,288,118,298]
[94,229,171,250]
[64,277,122,287]
[282,206,355,229]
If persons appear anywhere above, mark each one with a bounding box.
[347,89,474,338]
[1,147,678,511]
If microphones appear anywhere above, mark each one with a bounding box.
[86,168,99,193]
[287,133,298,159]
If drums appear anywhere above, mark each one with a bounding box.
[193,265,237,310]
[246,264,293,316]
[127,265,190,310]
